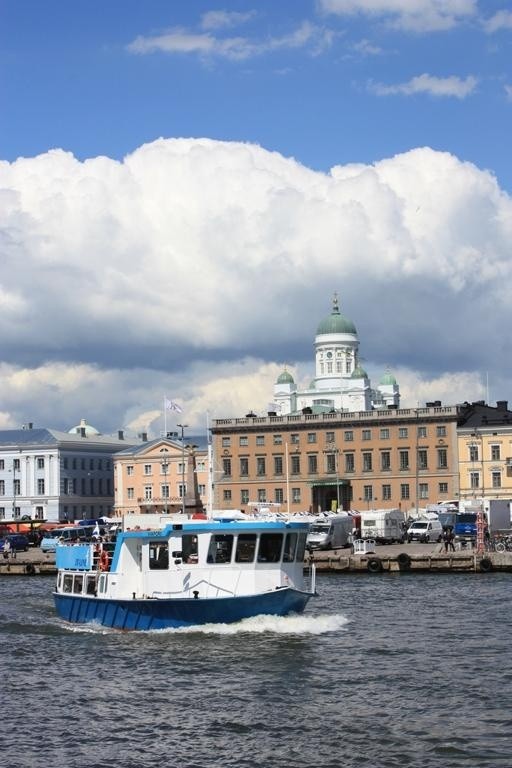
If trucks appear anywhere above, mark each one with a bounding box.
[439,512,489,543]
[351,509,405,545]
[306,516,354,550]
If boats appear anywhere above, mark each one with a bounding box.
[55,517,319,631]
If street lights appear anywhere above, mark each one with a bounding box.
[323,444,340,514]
[178,423,188,513]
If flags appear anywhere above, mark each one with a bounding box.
[165,395,184,414]
[331,499,338,514]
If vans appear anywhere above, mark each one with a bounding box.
[407,520,442,543]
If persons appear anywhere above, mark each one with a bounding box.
[3,538,13,558]
[58,532,115,547]
[442,527,457,551]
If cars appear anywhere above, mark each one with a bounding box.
[0,519,121,553]
